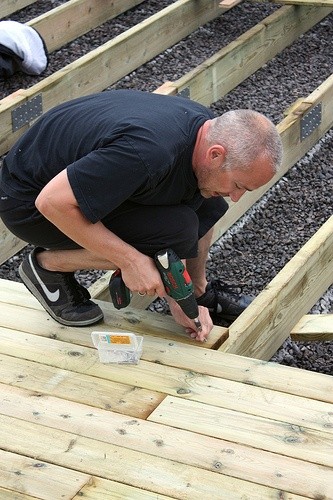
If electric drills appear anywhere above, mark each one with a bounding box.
[110,250,207,343]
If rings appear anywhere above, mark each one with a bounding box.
[137,291,146,297]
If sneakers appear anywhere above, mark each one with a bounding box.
[18,247,103,326]
[196,280,255,322]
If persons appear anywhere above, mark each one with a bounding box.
[1,90,281,342]
